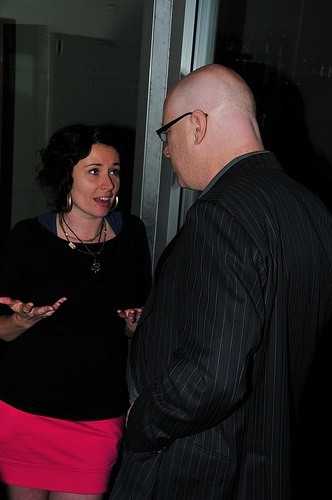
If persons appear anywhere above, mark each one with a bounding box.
[0,124,152,500]
[106,63,332,500]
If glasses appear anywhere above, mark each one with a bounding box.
[156,112,208,143]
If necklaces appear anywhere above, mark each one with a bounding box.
[57,209,108,275]
